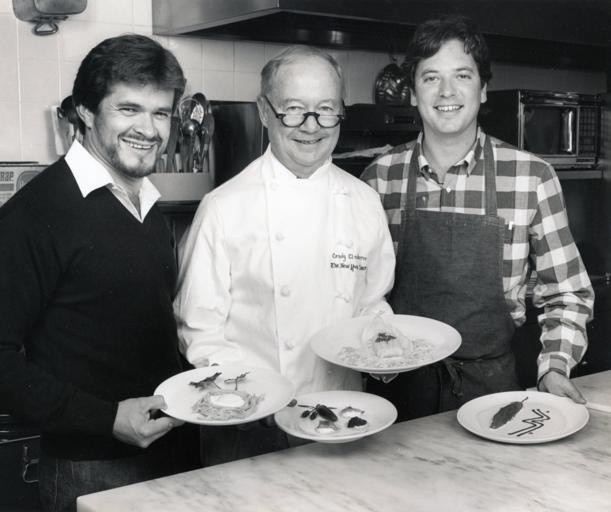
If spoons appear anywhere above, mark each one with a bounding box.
[174,91,212,172]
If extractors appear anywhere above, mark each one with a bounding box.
[148,0,573,71]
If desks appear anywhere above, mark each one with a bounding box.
[76,368,611,512]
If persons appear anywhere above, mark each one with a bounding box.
[354,16,595,427]
[172,47,395,471]
[0,34,180,512]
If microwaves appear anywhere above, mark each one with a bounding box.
[478,88,610,171]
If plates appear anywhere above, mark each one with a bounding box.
[456,389,590,444]
[152,365,297,428]
[273,389,399,446]
[308,313,466,375]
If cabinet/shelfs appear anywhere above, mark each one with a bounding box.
[210,100,603,189]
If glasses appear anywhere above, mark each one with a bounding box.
[263,95,346,128]
